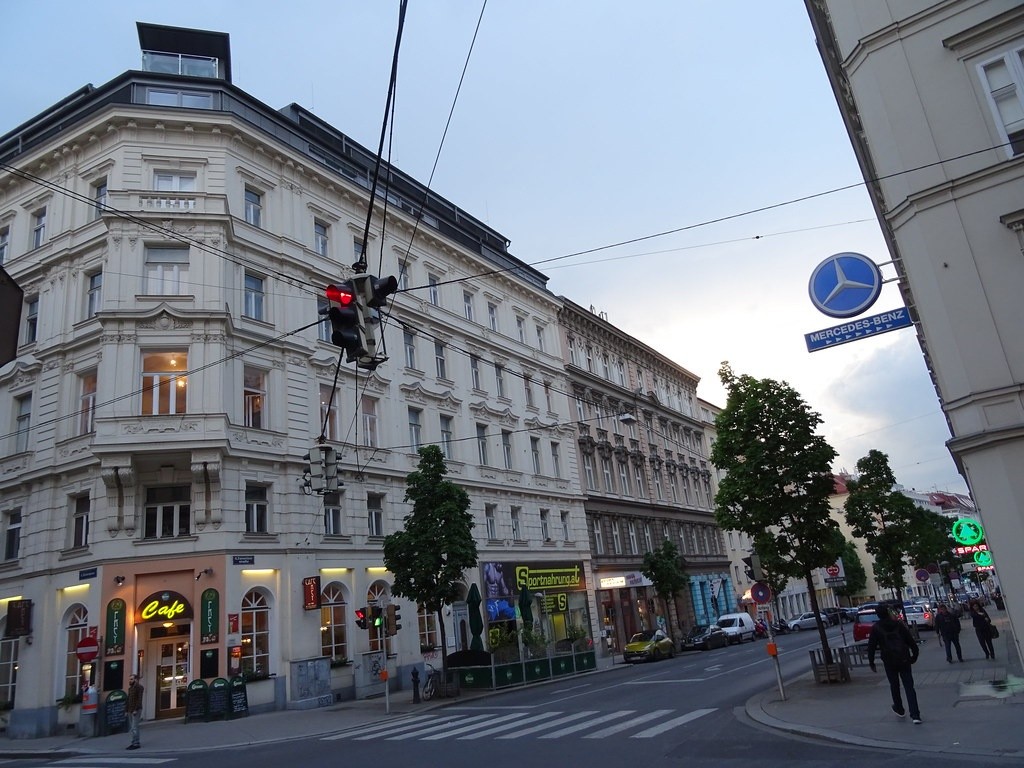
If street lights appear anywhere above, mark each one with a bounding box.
[938,561,954,609]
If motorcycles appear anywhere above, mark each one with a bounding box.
[755,618,790,639]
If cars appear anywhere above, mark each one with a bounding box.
[624,628,676,663]
[787,607,858,631]
[853,591,991,642]
[681,625,733,651]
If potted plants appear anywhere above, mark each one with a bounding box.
[243,668,269,683]
[421,644,434,655]
[56,692,82,724]
[329,655,348,669]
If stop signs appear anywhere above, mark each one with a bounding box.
[76,637,99,662]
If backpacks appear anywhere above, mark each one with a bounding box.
[875,623,909,663]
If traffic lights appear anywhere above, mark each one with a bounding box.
[354,608,367,629]
[326,283,368,363]
[394,604,402,634]
[372,606,383,627]
[303,448,323,490]
[327,451,344,490]
[366,275,398,307]
[742,556,755,581]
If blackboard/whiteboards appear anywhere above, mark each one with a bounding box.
[184,679,208,718]
[209,678,230,714]
[105,690,129,728]
[230,676,249,714]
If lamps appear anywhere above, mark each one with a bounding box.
[619,412,636,426]
[163,622,174,628]
[195,568,213,581]
[114,575,125,587]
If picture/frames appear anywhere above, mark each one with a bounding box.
[252,396,260,414]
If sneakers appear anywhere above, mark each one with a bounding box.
[891,704,906,717]
[912,713,922,724]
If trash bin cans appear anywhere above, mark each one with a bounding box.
[81,685,98,714]
[995,597,1005,610]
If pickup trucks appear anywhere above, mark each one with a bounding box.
[716,612,757,644]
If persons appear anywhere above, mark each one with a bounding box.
[971,603,995,660]
[935,603,964,663]
[125,674,144,750]
[867,602,924,724]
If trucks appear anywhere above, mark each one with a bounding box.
[605,626,616,650]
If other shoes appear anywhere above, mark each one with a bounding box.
[958,658,963,662]
[986,652,989,659]
[126,745,140,750]
[947,657,953,664]
[991,653,995,660]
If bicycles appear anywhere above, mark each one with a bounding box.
[422,663,442,701]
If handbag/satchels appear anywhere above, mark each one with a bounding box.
[988,624,999,638]
